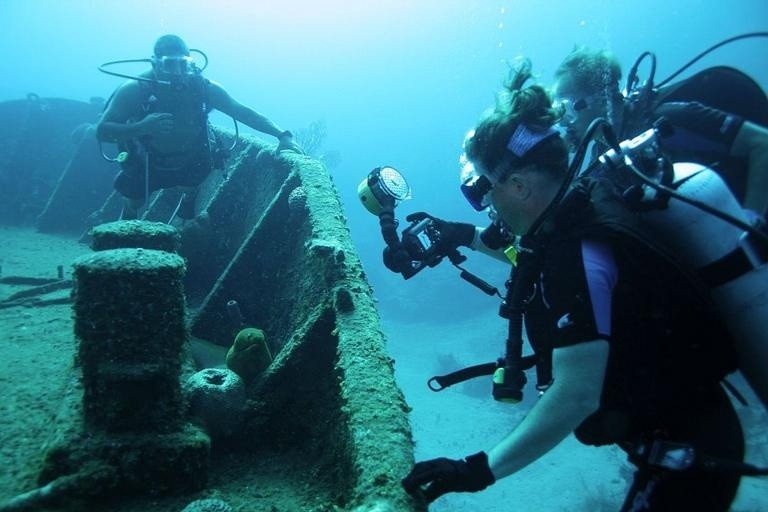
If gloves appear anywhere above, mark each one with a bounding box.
[406,211,475,267]
[401,451,495,502]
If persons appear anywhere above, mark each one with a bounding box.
[549,44,768,219]
[402,55,745,512]
[97,34,307,220]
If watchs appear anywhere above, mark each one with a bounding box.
[277,130,293,139]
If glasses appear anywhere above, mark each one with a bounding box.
[551,90,604,129]
[460,121,561,212]
[157,56,192,75]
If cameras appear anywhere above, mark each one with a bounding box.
[383,218,445,280]
